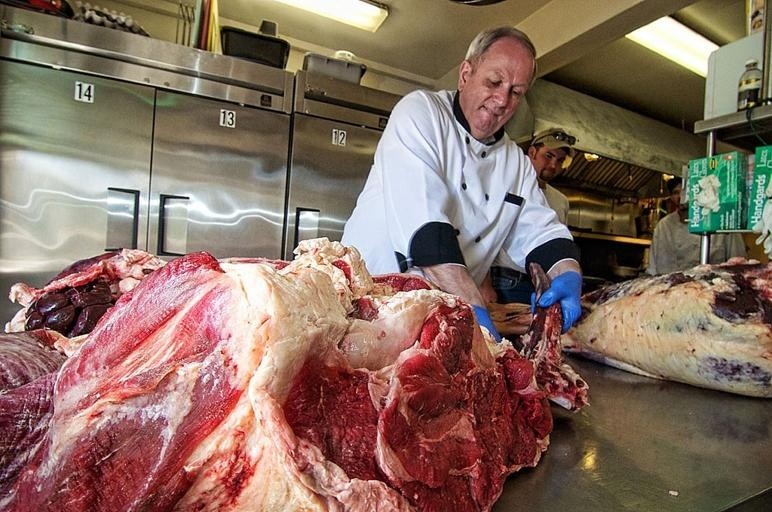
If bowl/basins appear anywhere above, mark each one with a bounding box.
[613,266,639,281]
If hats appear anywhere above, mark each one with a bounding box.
[531,127,576,159]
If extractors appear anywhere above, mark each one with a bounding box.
[530,78,705,208]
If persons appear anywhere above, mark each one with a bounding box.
[649,177,747,275]
[340,25,583,342]
[478,127,576,306]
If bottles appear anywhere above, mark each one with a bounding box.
[737,59,765,112]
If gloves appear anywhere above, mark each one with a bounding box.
[469,304,501,343]
[529,270,582,336]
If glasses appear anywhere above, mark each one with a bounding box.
[535,131,576,146]
